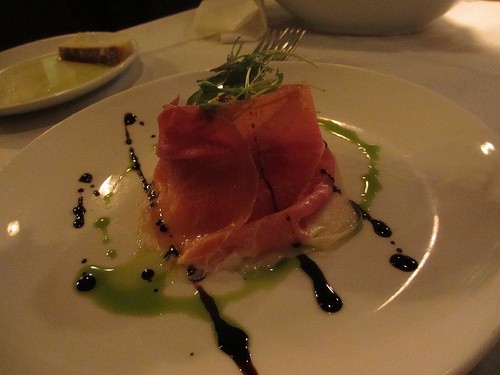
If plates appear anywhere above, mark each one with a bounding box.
[0,32,139,116]
[1,61,500,375]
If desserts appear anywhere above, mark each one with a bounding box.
[59,31,134,66]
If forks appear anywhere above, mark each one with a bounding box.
[246,27,282,63]
[272,27,306,60]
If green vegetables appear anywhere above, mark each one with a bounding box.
[185,35,326,123]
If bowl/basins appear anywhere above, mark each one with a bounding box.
[277,0,462,35]
